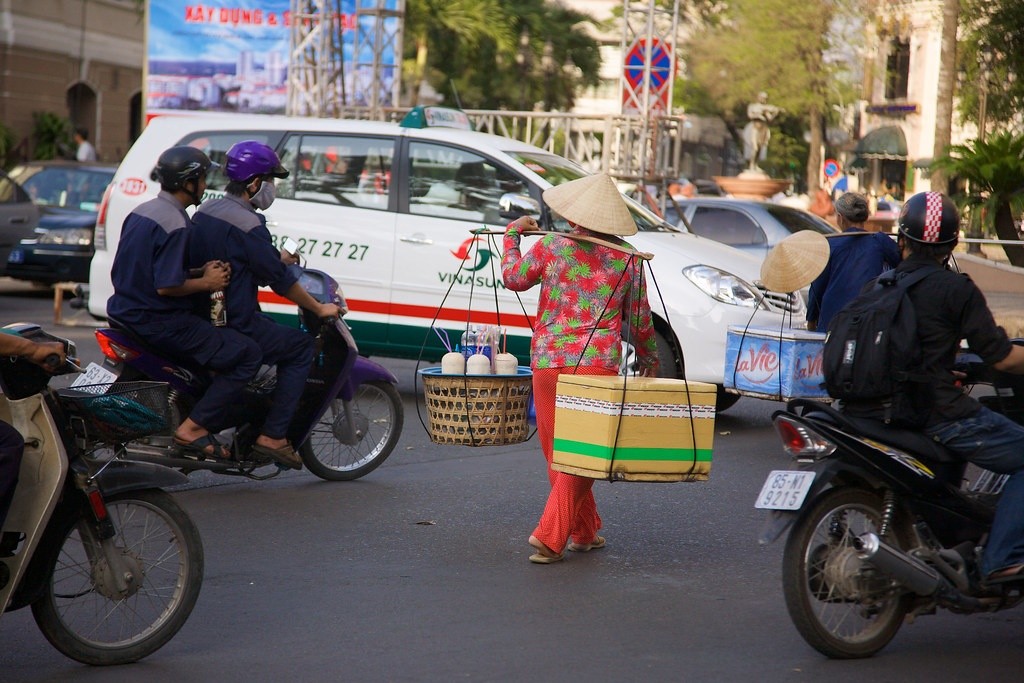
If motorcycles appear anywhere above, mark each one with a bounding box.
[0,318,207,667]
[754,336,1024,661]
[65,236,405,484]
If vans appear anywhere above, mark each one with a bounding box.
[85,111,809,418]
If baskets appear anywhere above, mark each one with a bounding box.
[57,380,172,445]
[421,369,532,447]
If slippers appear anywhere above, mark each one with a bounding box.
[252,437,302,470]
[986,565,1024,585]
[568,535,606,552]
[174,432,230,460]
[530,550,565,564]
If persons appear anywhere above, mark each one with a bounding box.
[859,192,1024,585]
[73,127,97,161]
[107,144,264,459]
[805,192,903,332]
[0,334,67,593]
[747,91,780,171]
[182,140,347,470]
[502,172,660,564]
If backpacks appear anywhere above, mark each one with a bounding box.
[822,266,948,431]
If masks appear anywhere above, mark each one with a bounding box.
[248,181,277,210]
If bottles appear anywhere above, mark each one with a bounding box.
[475,324,492,366]
[210,290,227,326]
[461,325,476,362]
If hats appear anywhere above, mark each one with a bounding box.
[761,230,830,293]
[542,172,638,236]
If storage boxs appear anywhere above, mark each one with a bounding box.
[550,375,717,481]
[723,325,834,403]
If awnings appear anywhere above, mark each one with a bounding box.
[856,125,908,162]
[865,103,918,114]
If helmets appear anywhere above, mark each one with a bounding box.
[898,192,960,243]
[157,146,219,188]
[223,141,289,183]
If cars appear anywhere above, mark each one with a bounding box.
[2,161,123,293]
[661,194,838,264]
